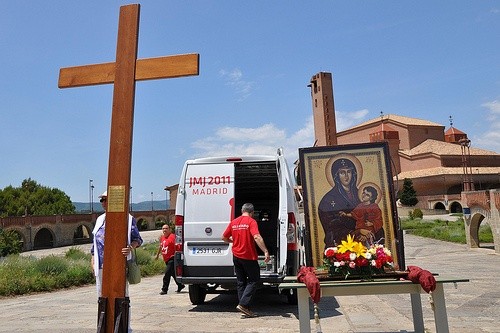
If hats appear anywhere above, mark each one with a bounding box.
[98,190,107,197]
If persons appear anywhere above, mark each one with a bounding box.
[153,224,186,295]
[89,191,144,333]
[221,202,270,318]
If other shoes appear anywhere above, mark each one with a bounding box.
[175,285,185,292]
[159,290,167,294]
[236,304,254,317]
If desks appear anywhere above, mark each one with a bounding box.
[279,278,470,333]
[283,272,439,333]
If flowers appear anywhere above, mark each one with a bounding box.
[323,231,394,280]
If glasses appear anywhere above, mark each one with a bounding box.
[100,198,106,202]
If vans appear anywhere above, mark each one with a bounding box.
[173,147,304,306]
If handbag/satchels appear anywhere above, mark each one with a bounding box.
[126,245,140,284]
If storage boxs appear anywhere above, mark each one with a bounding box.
[258,255,276,273]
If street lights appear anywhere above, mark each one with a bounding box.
[476,168,482,190]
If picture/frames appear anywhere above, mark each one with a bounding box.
[298,139,406,271]
